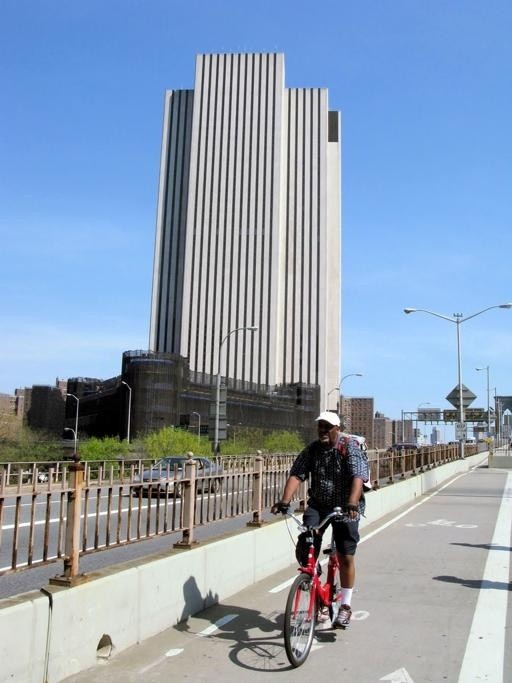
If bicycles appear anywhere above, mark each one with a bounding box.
[269,502,359,667]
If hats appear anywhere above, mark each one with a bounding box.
[315,412,340,427]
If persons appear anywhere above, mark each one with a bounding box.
[270,411,370,628]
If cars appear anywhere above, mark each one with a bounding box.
[130,454,224,499]
[384,443,421,465]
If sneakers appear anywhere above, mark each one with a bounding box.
[332,608,352,629]
[315,563,322,577]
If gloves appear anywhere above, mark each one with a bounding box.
[270,502,290,516]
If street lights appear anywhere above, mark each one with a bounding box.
[192,411,200,447]
[121,380,132,444]
[339,373,363,417]
[63,393,79,454]
[213,327,258,456]
[403,303,512,461]
[226,423,242,445]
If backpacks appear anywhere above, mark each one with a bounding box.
[348,434,372,492]
